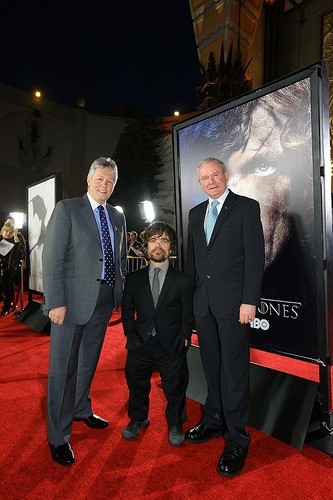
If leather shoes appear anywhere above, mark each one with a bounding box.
[186,422,225,443]
[0,308,8,316]
[75,414,109,429]
[217,441,249,477]
[47,441,75,466]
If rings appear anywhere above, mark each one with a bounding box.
[249,319,252,320]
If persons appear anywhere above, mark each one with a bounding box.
[180,77,317,359]
[126,230,143,257]
[0,222,20,315]
[184,157,265,476]
[4,217,27,310]
[41,157,125,466]
[121,222,194,446]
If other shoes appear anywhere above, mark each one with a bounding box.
[168,424,185,446]
[122,418,150,440]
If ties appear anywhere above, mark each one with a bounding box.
[205,200,220,245]
[97,205,115,287]
[151,268,161,338]
